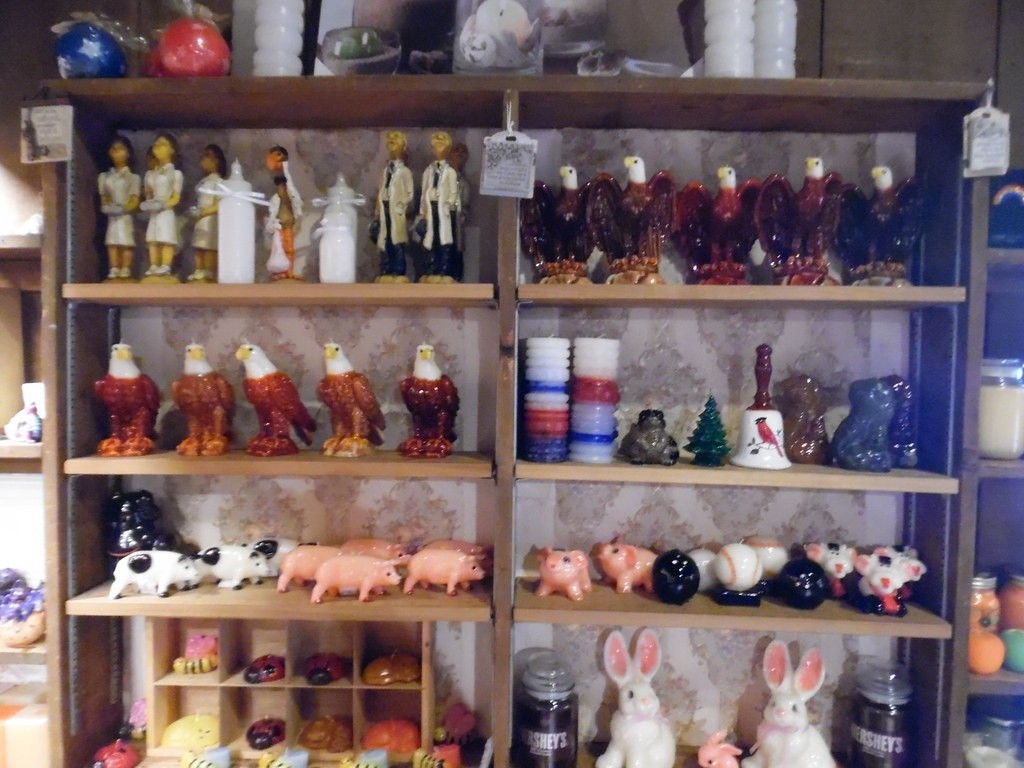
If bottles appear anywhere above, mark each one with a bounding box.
[846,661,914,768]
[516,653,578,768]
[999,574,1024,631]
[325,175,357,240]
[319,197,355,284]
[971,570,1001,637]
[219,158,255,285]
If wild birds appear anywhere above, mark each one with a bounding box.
[171,342,317,458]
[521,154,929,285]
[92,344,162,458]
[398,342,461,457]
[317,341,386,457]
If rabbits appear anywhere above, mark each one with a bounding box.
[592,628,676,768]
[694,729,744,768]
[742,638,838,767]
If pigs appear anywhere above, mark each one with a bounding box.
[107,527,490,604]
[536,536,928,619]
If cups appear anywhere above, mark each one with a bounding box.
[982,359,1024,459]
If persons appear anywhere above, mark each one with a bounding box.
[138,133,183,277]
[264,146,307,279]
[368,131,468,286]
[98,137,142,278]
[184,143,228,281]
